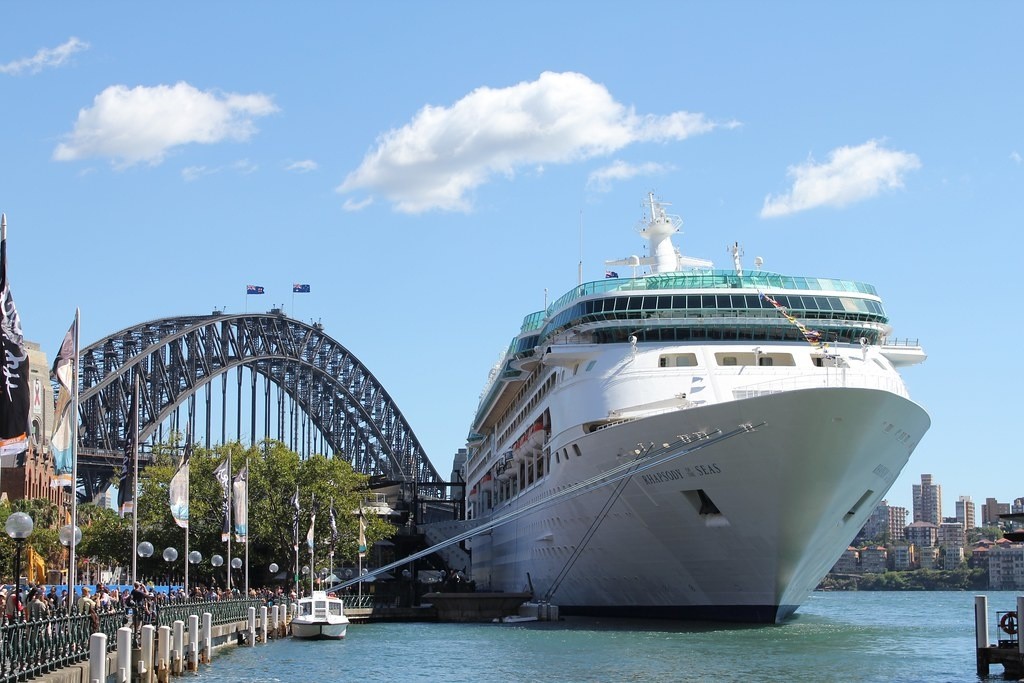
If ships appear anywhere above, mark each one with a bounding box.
[415,185,937,631]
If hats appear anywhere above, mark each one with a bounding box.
[82,586,91,593]
[36,589,46,597]
[10,585,21,593]
[0,592,6,599]
[135,582,141,587]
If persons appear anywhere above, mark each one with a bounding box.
[0,581,307,638]
[606,270,618,278]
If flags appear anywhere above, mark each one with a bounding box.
[117,386,133,518]
[232,462,246,542]
[358,511,367,558]
[290,491,300,551]
[212,456,230,541]
[759,292,828,350]
[293,283,310,293]
[0,239,31,455]
[49,314,75,488]
[303,501,315,554]
[169,411,192,528]
[327,506,337,555]
[247,285,265,294]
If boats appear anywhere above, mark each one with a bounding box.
[287,586,352,642]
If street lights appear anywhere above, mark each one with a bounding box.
[232,558,242,598]
[136,541,154,589]
[211,555,224,602]
[162,546,178,607]
[6,511,33,657]
[189,551,202,601]
[362,569,368,592]
[58,525,82,618]
[303,564,307,602]
[269,563,280,602]
[321,566,327,590]
